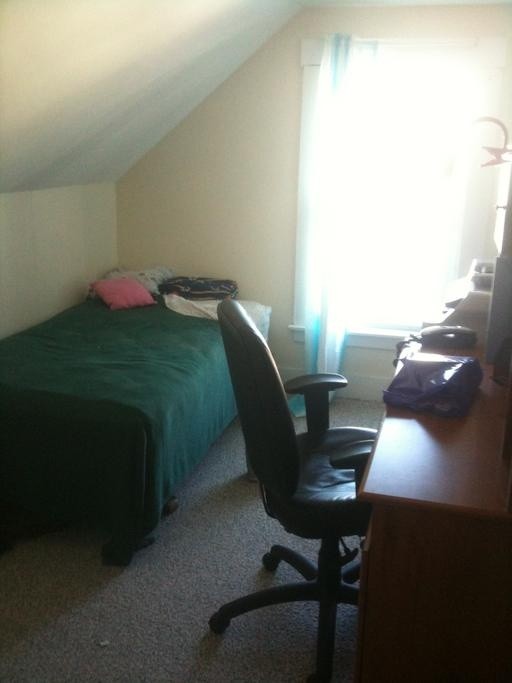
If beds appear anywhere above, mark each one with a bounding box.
[0,297,272,567]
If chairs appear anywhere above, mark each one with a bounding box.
[208,298,379,683]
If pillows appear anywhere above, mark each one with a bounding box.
[91,268,239,320]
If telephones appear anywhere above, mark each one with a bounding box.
[416,326,476,352]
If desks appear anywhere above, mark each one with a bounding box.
[356,326,512,682]
